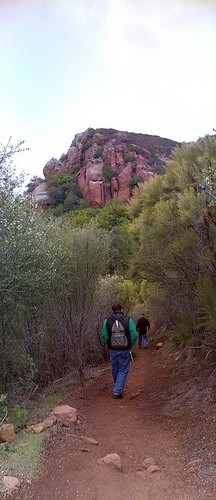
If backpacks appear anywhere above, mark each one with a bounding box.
[110,314,128,349]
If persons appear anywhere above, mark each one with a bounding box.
[136,313,150,348]
[102,304,137,400]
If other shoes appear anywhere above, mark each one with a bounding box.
[144,347,147,349]
[113,394,124,399]
